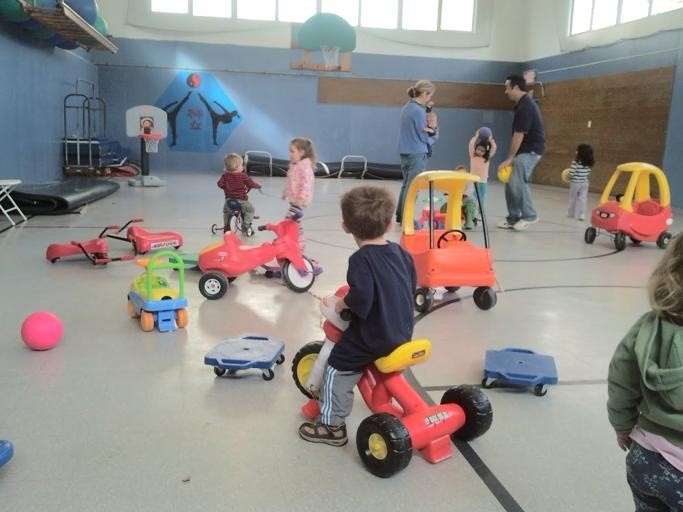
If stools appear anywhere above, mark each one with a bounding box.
[1,177,28,228]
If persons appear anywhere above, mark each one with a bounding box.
[423,101,438,159]
[606,229,683,512]
[567,144,594,220]
[217,153,262,233]
[468,129,497,221]
[443,165,476,230]
[497,76,546,232]
[395,80,440,227]
[282,138,315,251]
[299,186,416,447]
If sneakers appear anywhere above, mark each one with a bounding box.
[299,423,347,446]
[497,219,512,229]
[513,218,539,230]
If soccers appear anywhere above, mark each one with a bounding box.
[497,165,511,182]
[22,312,62,350]
[562,169,571,183]
